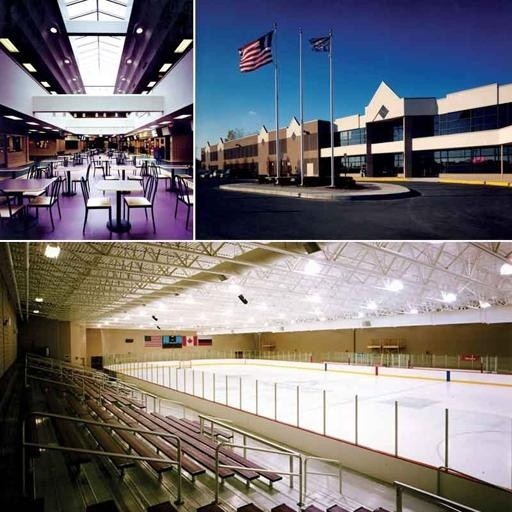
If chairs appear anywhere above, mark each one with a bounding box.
[0,155,192,240]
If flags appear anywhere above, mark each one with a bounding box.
[236,28,275,73]
[144,335,162,348]
[163,335,182,348]
[308,35,330,53]
[198,335,212,346]
[183,335,197,347]
